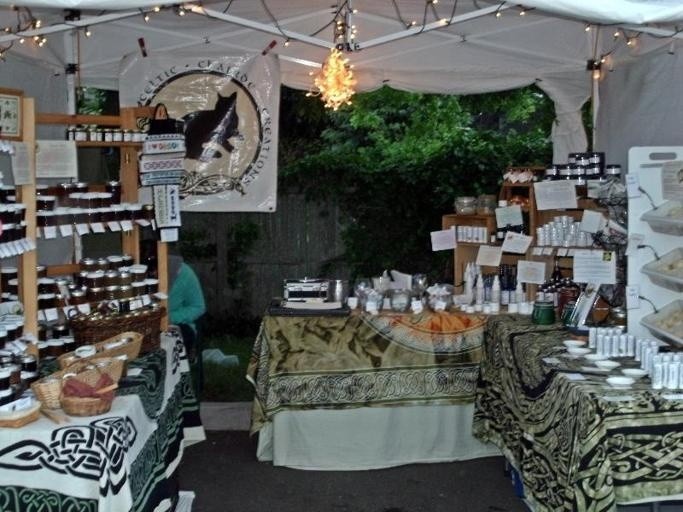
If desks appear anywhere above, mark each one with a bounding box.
[1,324,206,512]
[471,309,682,511]
[244,303,519,472]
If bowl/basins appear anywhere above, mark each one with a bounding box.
[566,347,592,357]
[585,353,608,363]
[595,360,621,372]
[621,368,648,380]
[605,377,635,387]
[563,340,586,348]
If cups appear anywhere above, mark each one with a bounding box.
[531,300,556,326]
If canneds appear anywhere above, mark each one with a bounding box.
[546,152,621,180]
[588,327,683,390]
[0,128,159,405]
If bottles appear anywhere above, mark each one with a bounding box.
[536,260,571,308]
[499,265,515,305]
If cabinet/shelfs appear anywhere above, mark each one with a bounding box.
[0,98,169,333]
[626,145,681,391]
[444,167,627,332]
[442,144,683,377]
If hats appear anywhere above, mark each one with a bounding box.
[140,240,158,270]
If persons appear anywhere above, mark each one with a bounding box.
[145,252,206,413]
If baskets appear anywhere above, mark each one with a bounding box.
[0,306,167,428]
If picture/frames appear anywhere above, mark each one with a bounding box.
[0,86,24,142]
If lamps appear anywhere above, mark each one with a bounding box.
[305,48,356,111]
